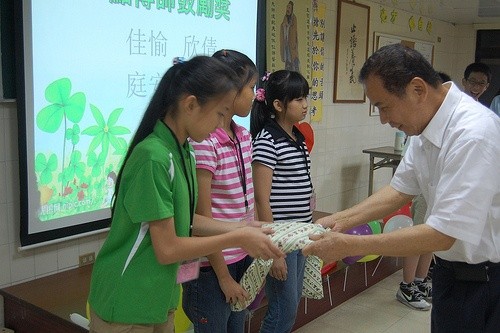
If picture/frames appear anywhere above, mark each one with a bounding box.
[332,0,372,105]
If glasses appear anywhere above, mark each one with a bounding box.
[467,78,489,87]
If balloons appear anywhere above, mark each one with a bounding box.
[321,201,414,274]
[173,285,191,333]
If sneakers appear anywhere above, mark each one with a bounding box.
[414,276,433,303]
[396,281,431,311]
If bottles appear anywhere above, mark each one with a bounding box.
[394,131,405,150]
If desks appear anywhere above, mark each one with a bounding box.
[361,145,404,198]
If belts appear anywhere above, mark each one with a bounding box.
[431,254,500,270]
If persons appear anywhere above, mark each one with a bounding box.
[183,49,257,333]
[302,44,500,333]
[87,57,285,333]
[398,72,453,309]
[490,92,500,117]
[461,62,492,101]
[249,71,314,333]
